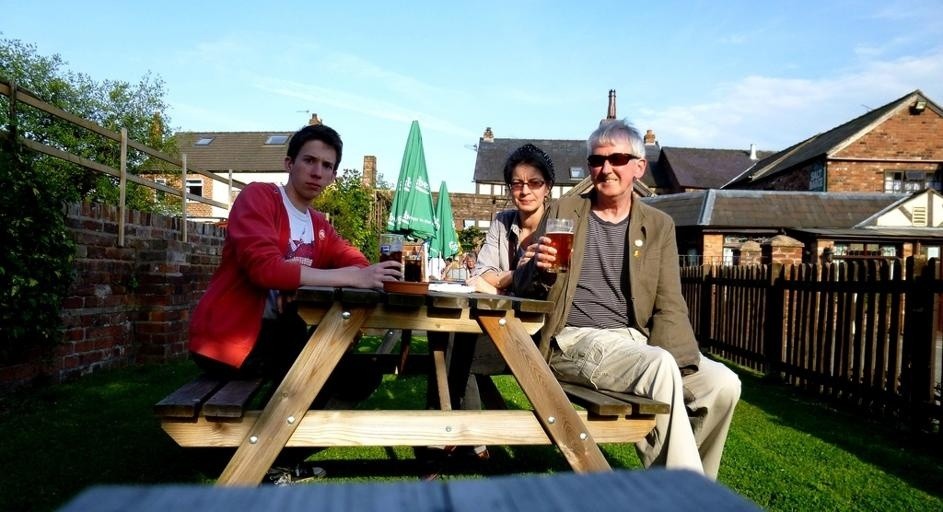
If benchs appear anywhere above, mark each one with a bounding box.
[153,368,268,448]
[556,380,671,445]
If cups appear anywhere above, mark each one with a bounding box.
[545,218,575,272]
[404,254,421,282]
[379,232,402,279]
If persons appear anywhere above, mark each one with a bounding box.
[512,119,743,483]
[418,143,555,480]
[185,124,402,488]
[428,253,477,283]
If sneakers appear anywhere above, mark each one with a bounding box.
[264,464,327,486]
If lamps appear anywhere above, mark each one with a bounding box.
[824,248,834,263]
[914,100,927,111]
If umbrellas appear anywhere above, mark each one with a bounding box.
[429,180,460,281]
[384,120,437,242]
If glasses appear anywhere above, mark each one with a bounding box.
[509,179,546,191]
[587,153,640,167]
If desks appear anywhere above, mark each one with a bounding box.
[55,466,765,512]
[210,283,614,486]
